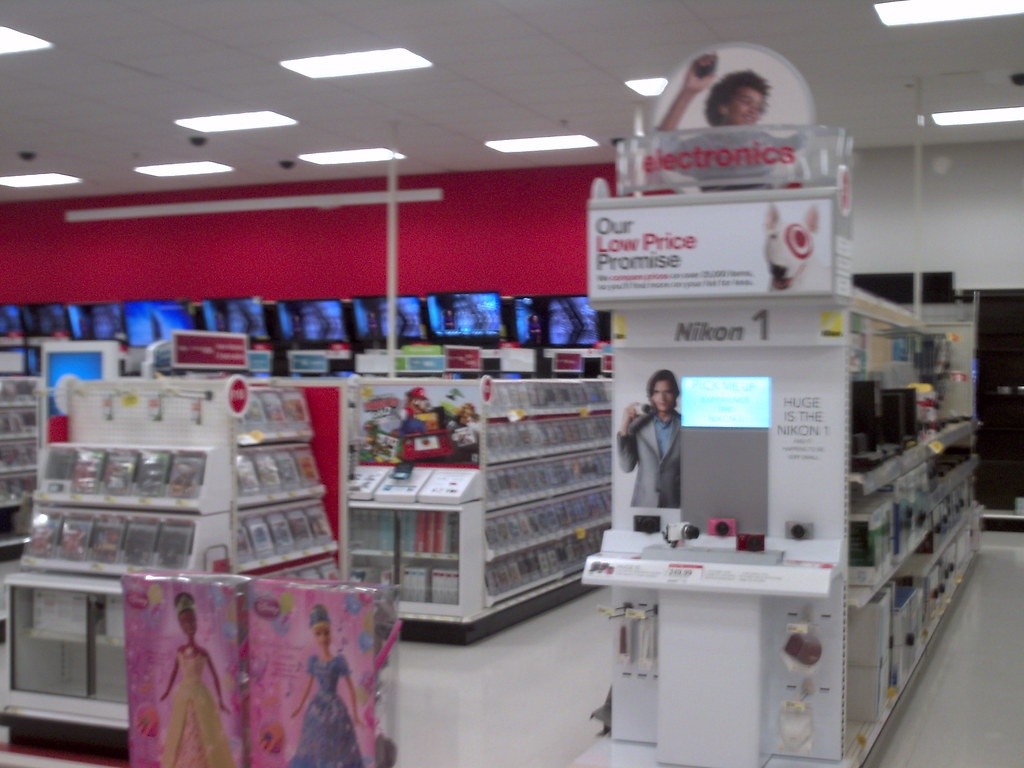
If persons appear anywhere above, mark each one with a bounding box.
[288,603,366,768]
[658,53,776,193]
[616,370,681,533]
[159,592,232,768]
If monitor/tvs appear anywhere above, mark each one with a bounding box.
[0,288,611,386]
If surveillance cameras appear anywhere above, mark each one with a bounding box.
[19,151,35,161]
[612,138,624,151]
[1012,73,1024,85]
[280,160,295,169]
[191,137,207,146]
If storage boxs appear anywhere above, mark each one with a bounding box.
[9,582,131,704]
[352,509,461,604]
[349,465,486,505]
[852,378,982,722]
[122,568,402,768]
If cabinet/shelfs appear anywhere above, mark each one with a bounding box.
[0,371,47,555]
[0,377,340,757]
[581,177,981,768]
[345,377,616,647]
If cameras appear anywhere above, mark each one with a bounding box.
[634,515,814,552]
[635,403,652,415]
[696,62,717,77]
